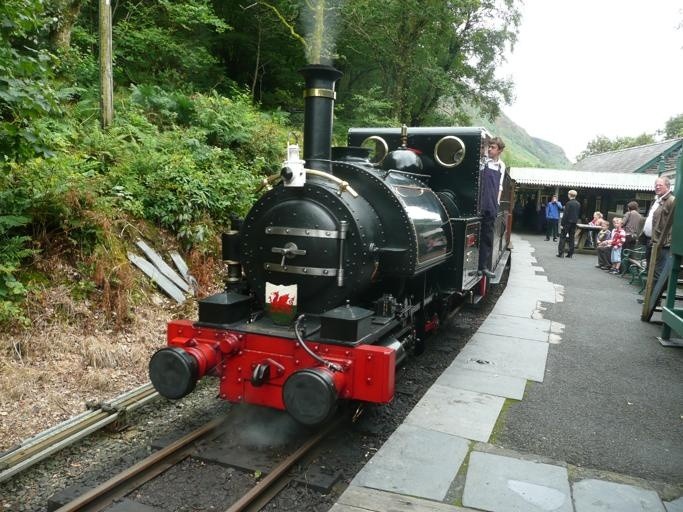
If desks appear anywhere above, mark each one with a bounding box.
[571,223,607,253]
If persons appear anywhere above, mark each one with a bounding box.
[514,189,643,275]
[637,178,675,306]
[479,137,506,278]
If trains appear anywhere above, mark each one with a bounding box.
[144,62,519,426]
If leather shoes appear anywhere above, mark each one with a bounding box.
[482,269,495,278]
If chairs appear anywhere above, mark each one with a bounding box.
[618,242,654,294]
